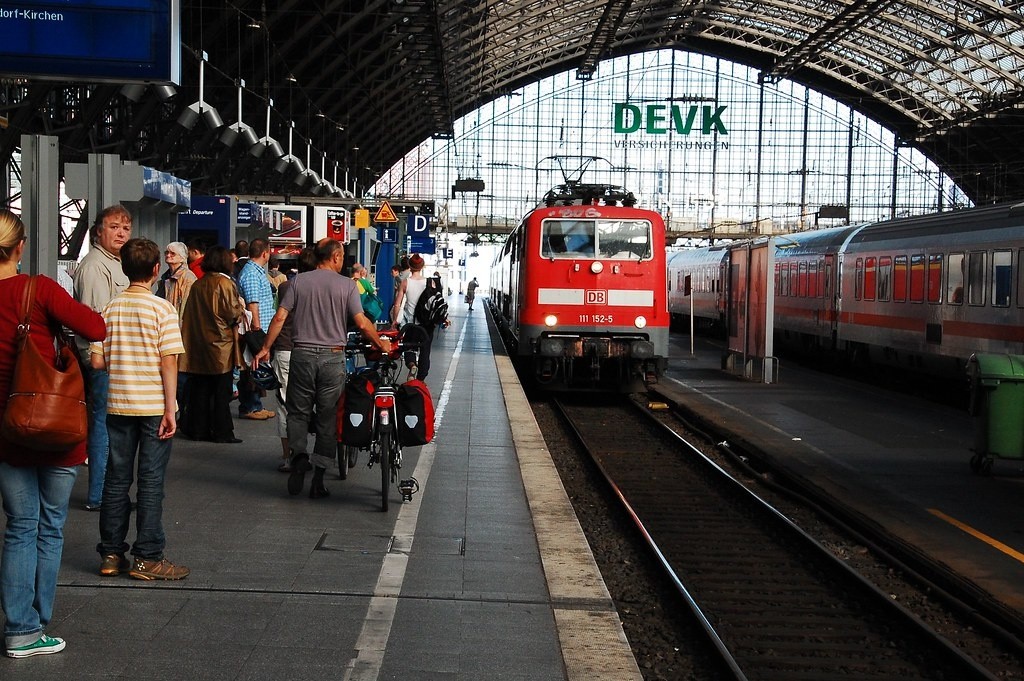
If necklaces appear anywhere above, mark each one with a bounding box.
[129,285,150,291]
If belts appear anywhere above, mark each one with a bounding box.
[294,345,343,350]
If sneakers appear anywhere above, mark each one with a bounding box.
[99,553,130,576]
[239,410,269,420]
[6,633,66,658]
[260,409,276,418]
[129,556,190,580]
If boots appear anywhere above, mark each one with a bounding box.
[407,362,418,381]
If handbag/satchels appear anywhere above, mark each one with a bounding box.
[336,369,376,447]
[464,296,469,303]
[4,324,87,446]
[362,295,384,323]
[396,379,435,446]
[245,329,266,354]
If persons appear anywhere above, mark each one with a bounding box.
[88,236,188,580]
[467,277,479,310]
[0,209,107,660]
[72,206,140,511]
[154,231,450,503]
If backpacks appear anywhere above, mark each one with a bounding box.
[414,278,448,324]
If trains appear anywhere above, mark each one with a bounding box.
[488,180,671,385]
[667,203,1024,364]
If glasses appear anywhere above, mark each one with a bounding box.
[164,251,178,255]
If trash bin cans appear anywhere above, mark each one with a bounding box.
[966,353,1024,460]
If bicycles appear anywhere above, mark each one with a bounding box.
[336,393,357,479]
[343,332,420,511]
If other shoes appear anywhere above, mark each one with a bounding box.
[468,308,474,310]
[310,485,330,498]
[215,431,243,443]
[278,458,289,471]
[288,458,306,495]
[130,500,138,510]
